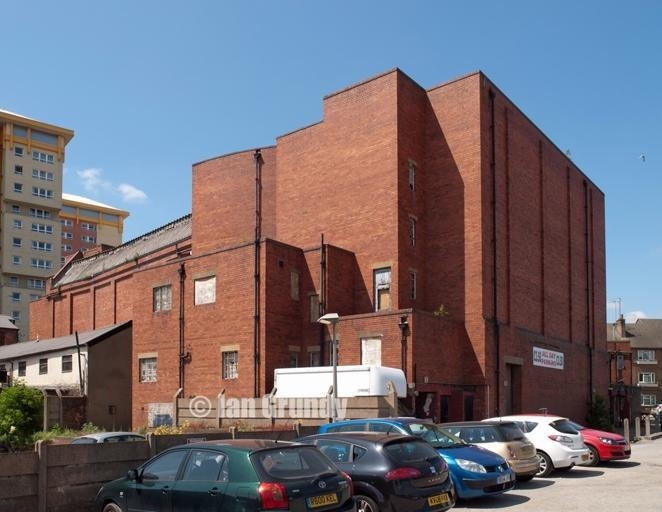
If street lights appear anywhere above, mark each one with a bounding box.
[317,312,339,398]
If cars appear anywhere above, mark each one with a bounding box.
[481,415,592,478]
[68,432,147,445]
[288,431,458,512]
[319,418,517,503]
[513,413,632,467]
[92,439,357,512]
[421,421,541,483]
